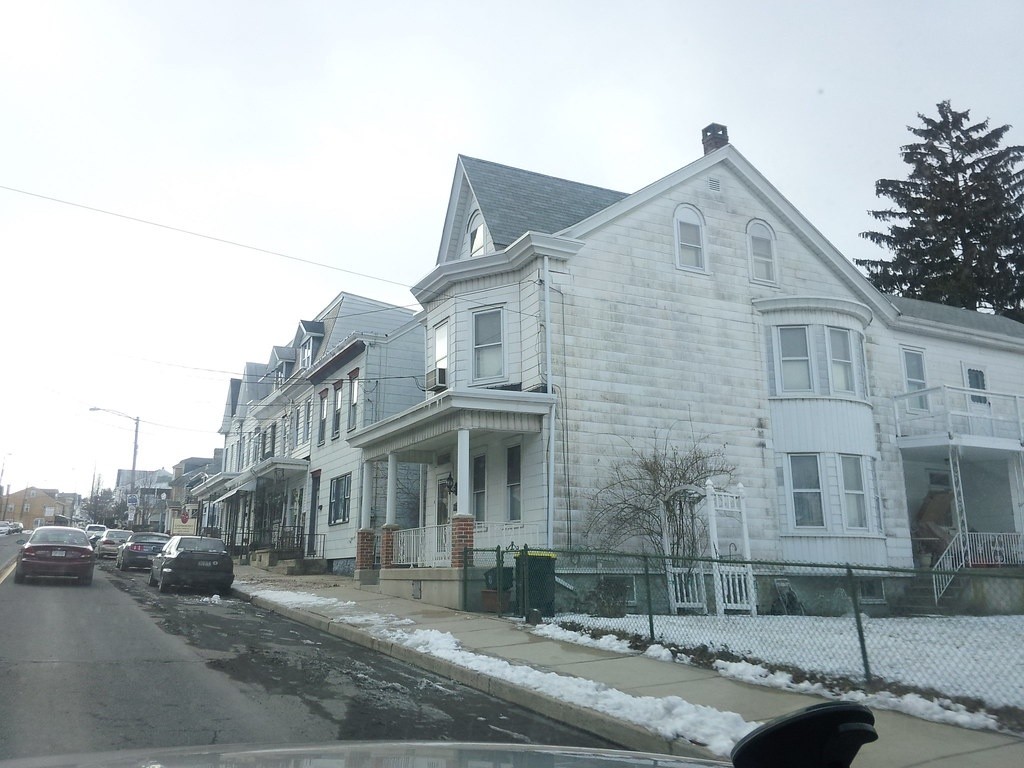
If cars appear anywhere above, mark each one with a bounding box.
[85,522,107,536]
[14,525,94,586]
[115,531,172,570]
[148,535,234,595]
[1,519,24,536]
[95,528,134,558]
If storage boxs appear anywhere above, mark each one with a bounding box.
[481,589,512,613]
[483,566,514,590]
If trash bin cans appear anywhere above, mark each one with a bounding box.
[514,548,557,618]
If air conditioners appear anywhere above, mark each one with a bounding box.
[425,368,446,391]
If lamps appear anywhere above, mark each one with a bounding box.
[446,472,454,495]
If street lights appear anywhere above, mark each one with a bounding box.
[88,406,140,505]
